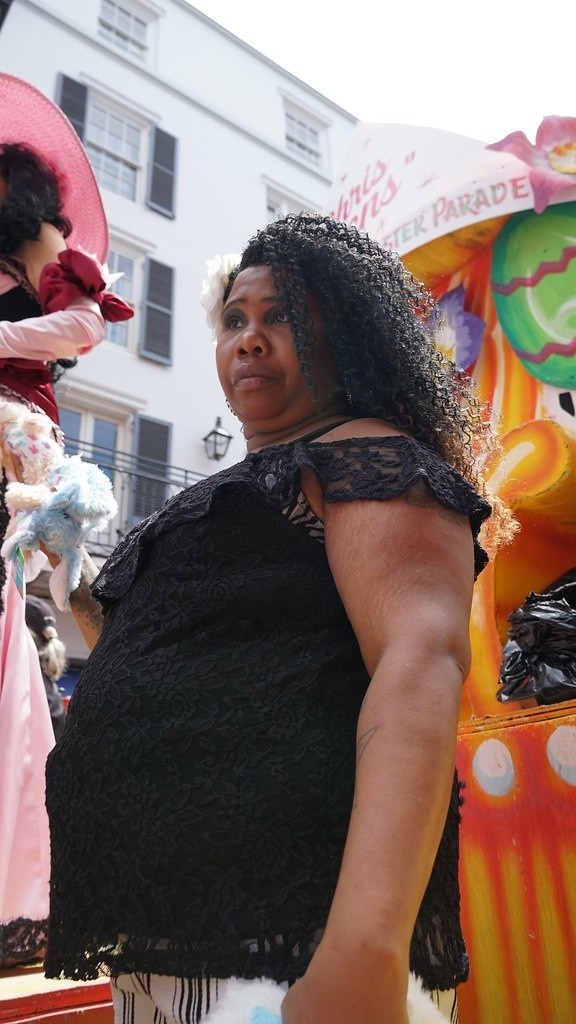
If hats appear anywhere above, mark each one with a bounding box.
[25,594,56,643]
[0,71,110,265]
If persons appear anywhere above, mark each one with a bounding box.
[0,73,134,966]
[4,213,520,1023]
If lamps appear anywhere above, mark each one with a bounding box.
[203,418,231,460]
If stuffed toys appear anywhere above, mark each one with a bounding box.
[0,393,118,612]
